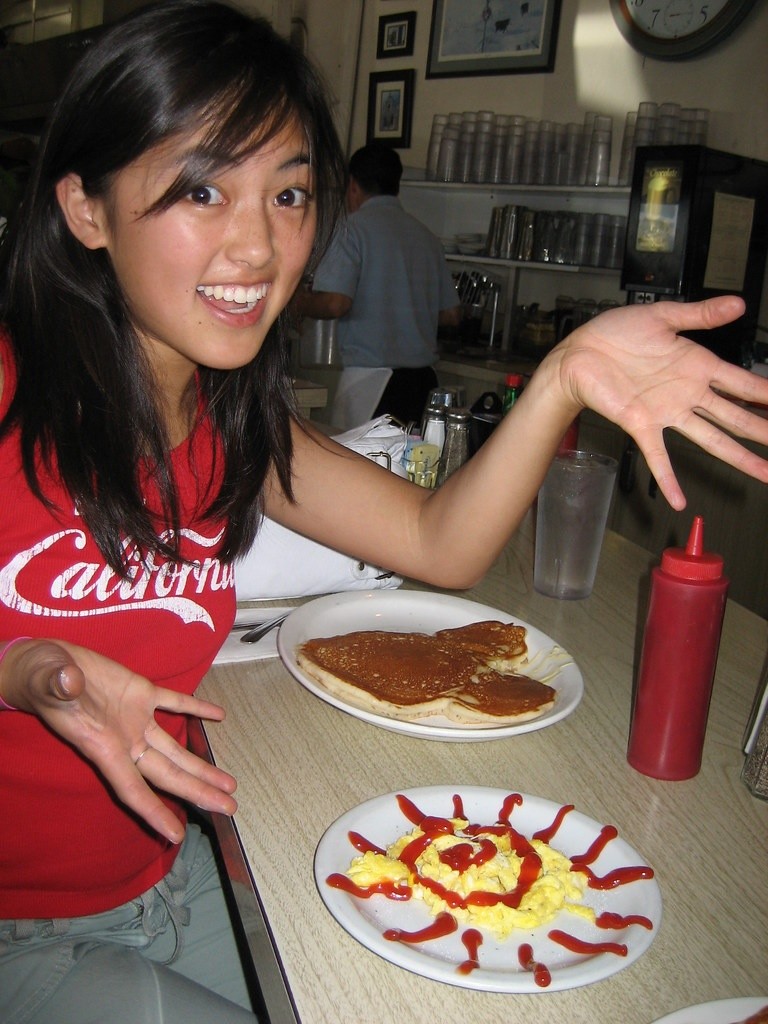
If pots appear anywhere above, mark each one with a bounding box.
[294,316,343,371]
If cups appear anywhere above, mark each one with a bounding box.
[425,101,711,188]
[533,450,620,600]
[485,206,627,269]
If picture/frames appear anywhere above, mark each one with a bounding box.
[376,10,416,60]
[425,0,562,81]
[366,68,414,147]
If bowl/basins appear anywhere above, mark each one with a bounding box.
[439,233,483,255]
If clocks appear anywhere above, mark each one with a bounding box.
[607,0,756,60]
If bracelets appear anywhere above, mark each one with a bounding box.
[0,637,37,710]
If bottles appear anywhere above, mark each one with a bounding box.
[626,516,730,782]
[421,404,473,490]
[502,375,523,417]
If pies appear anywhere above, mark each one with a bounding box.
[294,612,558,725]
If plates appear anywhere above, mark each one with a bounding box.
[277,589,583,742]
[649,996,768,1024]
[315,785,662,993]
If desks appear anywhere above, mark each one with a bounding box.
[187,502,767,1023]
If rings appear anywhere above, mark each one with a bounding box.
[134,745,153,765]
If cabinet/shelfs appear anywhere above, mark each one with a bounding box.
[403,179,632,404]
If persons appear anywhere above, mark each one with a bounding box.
[289,145,463,431]
[0,0,767,1024]
[381,96,394,130]
[389,24,405,47]
